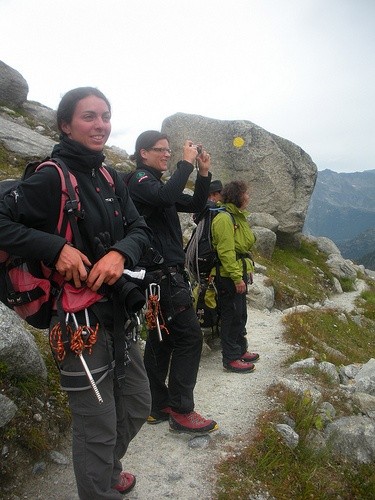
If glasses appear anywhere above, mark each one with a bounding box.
[144,147,172,153]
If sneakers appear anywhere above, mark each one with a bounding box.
[223,357,255,373]
[168,408,218,437]
[110,472,136,495]
[147,408,171,424]
[240,351,259,363]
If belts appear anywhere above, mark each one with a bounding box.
[146,266,185,274]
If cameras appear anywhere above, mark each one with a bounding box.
[190,145,201,154]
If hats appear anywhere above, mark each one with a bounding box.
[209,180,223,193]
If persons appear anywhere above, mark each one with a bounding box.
[126,130,217,434]
[0,87,157,500]
[186,179,224,350]
[209,180,261,374]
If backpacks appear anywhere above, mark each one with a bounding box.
[192,280,220,328]
[183,203,240,284]
[0,157,116,330]
